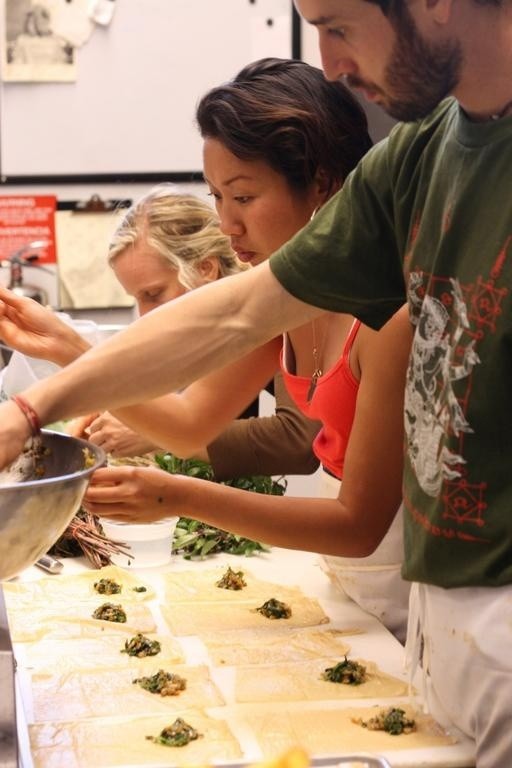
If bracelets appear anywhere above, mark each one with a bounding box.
[11,393,42,437]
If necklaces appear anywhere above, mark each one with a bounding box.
[491,94,512,120]
[307,312,331,407]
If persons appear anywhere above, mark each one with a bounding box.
[2,57,410,646]
[2,3,512,764]
[68,193,331,471]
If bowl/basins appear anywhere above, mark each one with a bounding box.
[0,418,104,578]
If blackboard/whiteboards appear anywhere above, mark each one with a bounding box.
[0,0,302,187]
